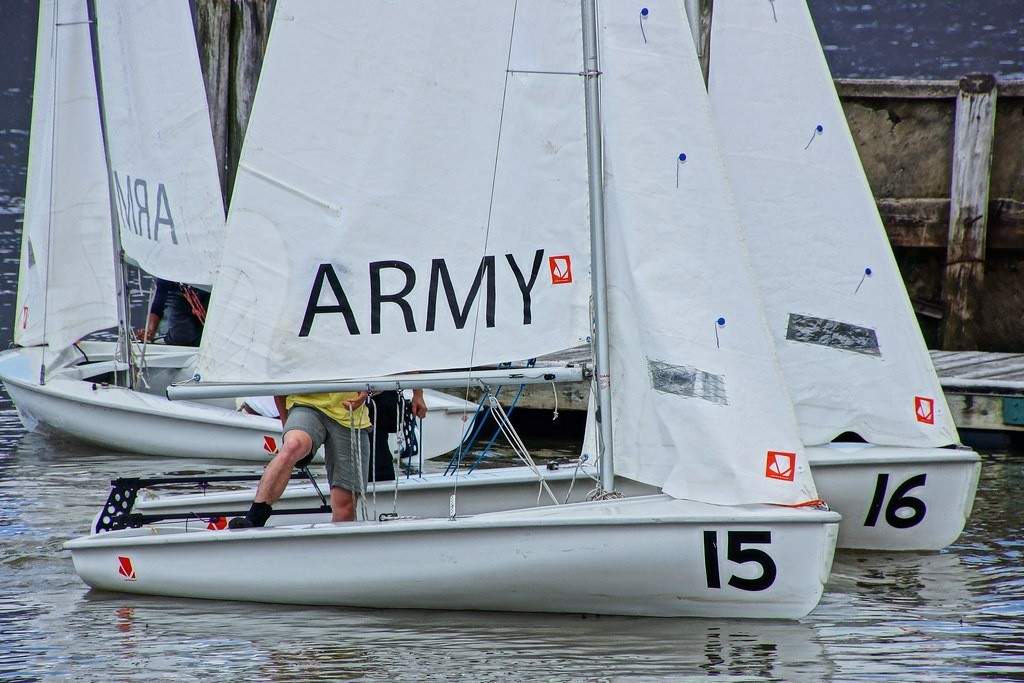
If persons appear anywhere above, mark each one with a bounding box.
[366,389,427,482]
[137,277,211,347]
[228,391,374,529]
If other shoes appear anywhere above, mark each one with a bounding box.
[229,518,254,529]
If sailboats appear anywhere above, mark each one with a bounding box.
[120,0,985,557]
[65,0,837,622]
[1,4,481,468]
